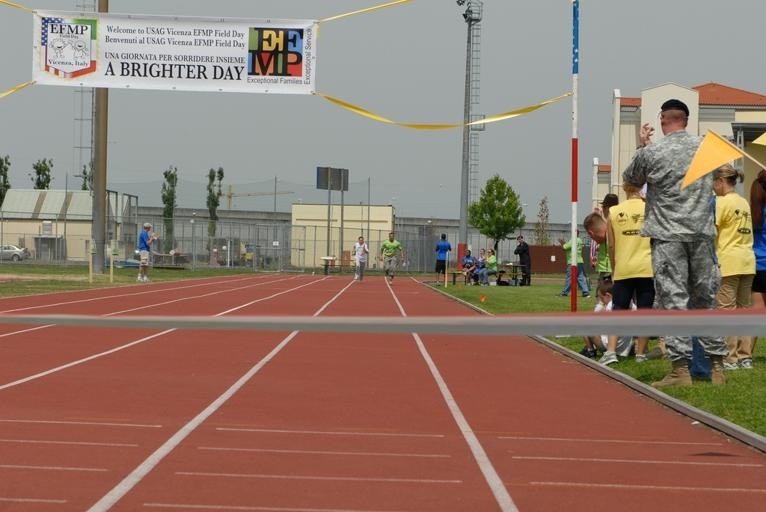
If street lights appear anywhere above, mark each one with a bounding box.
[423,219,433,273]
[438,183,443,217]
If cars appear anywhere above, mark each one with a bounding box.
[0,244,30,261]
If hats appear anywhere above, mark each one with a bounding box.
[599,193,619,206]
[144,222,153,228]
[660,98,689,116]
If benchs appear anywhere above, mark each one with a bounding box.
[448,263,527,286]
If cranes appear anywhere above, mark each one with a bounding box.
[218,184,295,209]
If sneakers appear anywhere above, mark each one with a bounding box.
[582,294,591,299]
[740,358,756,370]
[518,283,531,286]
[558,293,568,296]
[579,343,596,359]
[723,361,740,371]
[467,282,490,287]
[598,352,619,367]
[137,277,151,282]
[634,354,647,363]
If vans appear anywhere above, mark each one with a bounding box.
[204,236,239,256]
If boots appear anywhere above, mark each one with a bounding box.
[645,335,668,360]
[711,354,727,385]
[651,358,692,388]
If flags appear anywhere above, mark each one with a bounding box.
[240,241,248,255]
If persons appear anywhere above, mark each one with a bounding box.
[20,246,25,251]
[380,232,403,284]
[496,271,510,286]
[461,248,498,287]
[353,236,369,282]
[136,223,157,282]
[514,236,531,286]
[432,233,452,286]
[244,249,253,270]
